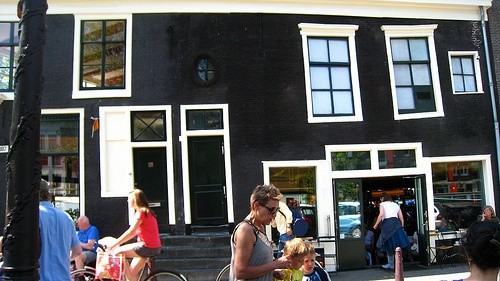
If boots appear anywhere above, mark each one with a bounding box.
[381,254,394,269]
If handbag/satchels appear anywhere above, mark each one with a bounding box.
[96,250,126,281]
[278,206,309,237]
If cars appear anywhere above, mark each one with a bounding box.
[338,202,361,239]
[298,205,316,236]
[434,204,443,231]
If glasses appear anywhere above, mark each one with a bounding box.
[260,204,280,214]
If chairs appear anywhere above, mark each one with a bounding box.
[425,230,467,269]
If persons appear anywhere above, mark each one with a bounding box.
[0,178,82,281]
[108,189,161,281]
[364,193,417,270]
[440,206,500,281]
[229,184,321,281]
[75,215,99,281]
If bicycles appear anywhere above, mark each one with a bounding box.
[215,247,332,281]
[70,242,188,281]
